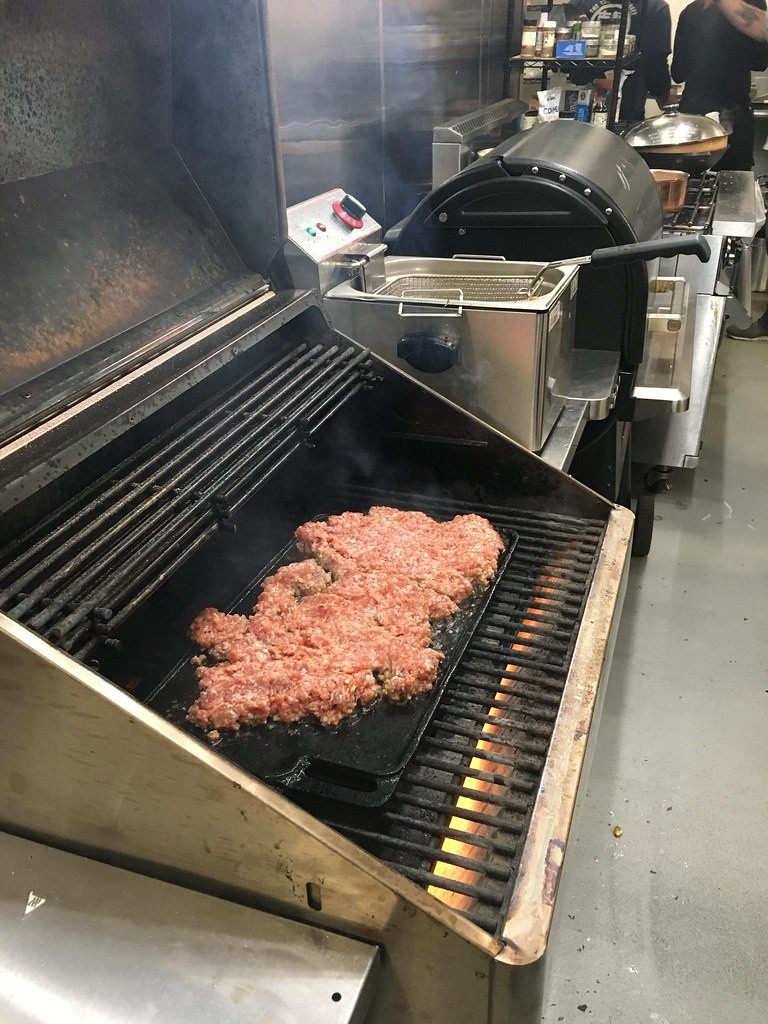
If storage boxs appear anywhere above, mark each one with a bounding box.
[556,40,586,58]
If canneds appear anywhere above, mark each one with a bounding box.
[534,21,572,58]
[574,103,590,123]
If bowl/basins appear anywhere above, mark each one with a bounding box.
[650,169,690,213]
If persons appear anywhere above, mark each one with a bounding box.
[727,309,768,341]
[671,0,768,171]
[563,0,678,137]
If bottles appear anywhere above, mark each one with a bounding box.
[520,11,636,59]
[593,97,608,129]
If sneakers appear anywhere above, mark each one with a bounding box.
[726,321,768,341]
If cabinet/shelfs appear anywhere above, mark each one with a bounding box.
[502,0,648,132]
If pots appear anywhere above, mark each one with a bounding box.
[620,103,733,175]
